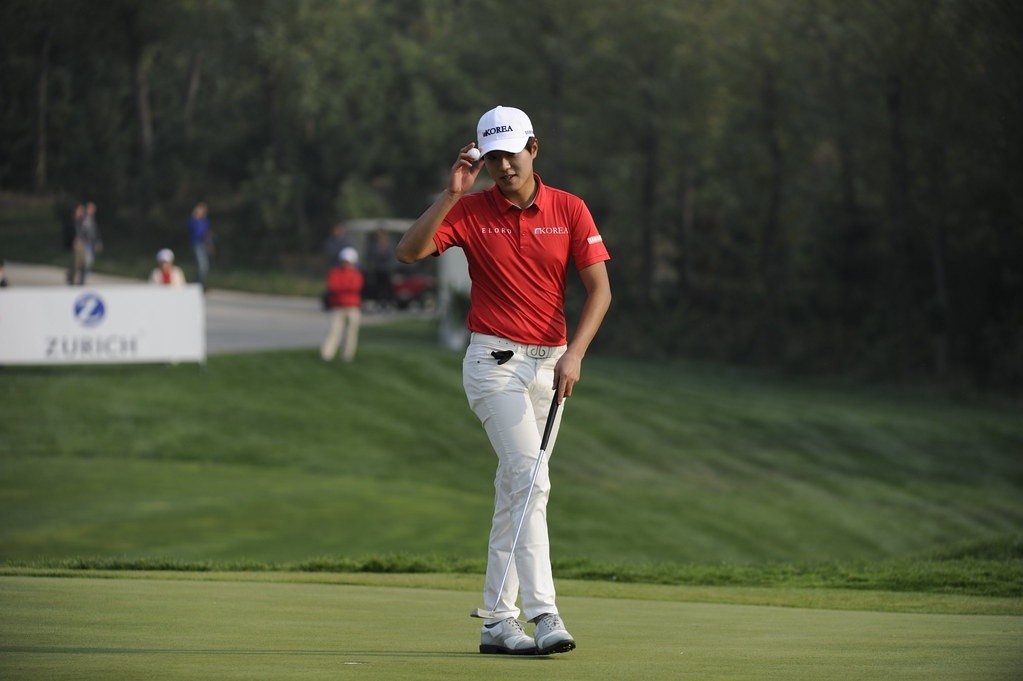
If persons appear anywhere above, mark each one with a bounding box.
[320,246,364,361]
[394,106,613,654]
[191,203,210,289]
[64,201,101,285]
[149,249,185,285]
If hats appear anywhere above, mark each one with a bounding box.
[476,105,534,160]
[157,248,175,263]
[339,248,359,264]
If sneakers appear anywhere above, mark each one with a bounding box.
[532,615,575,654]
[478,618,538,655]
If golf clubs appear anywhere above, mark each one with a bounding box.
[471,375,561,618]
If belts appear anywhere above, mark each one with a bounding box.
[471,333,566,357]
